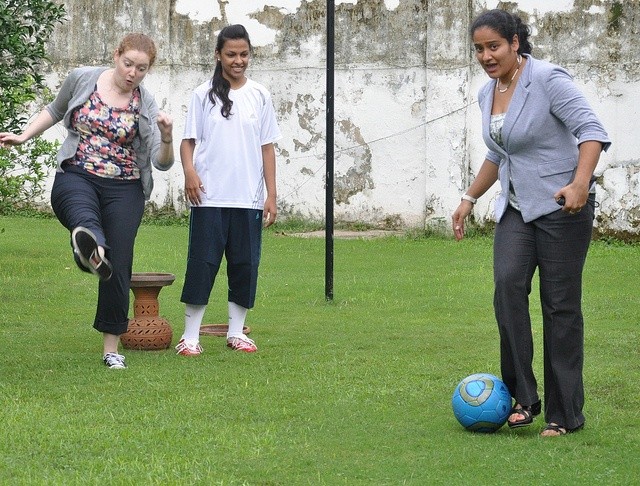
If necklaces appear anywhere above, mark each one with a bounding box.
[495,55,522,94]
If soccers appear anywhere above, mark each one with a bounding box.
[451,373,511,434]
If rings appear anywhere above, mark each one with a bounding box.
[200,183,204,188]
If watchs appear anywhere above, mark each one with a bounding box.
[460,191,478,204]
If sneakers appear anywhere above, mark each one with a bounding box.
[226,332,257,352]
[102,352,128,370]
[174,334,204,356]
[71,226,114,282]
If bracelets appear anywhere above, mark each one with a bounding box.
[161,138,173,144]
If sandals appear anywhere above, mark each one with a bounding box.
[506,398,541,429]
[539,422,584,437]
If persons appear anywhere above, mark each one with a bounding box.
[1,33,175,370]
[176,24,279,357]
[451,9,612,438]
